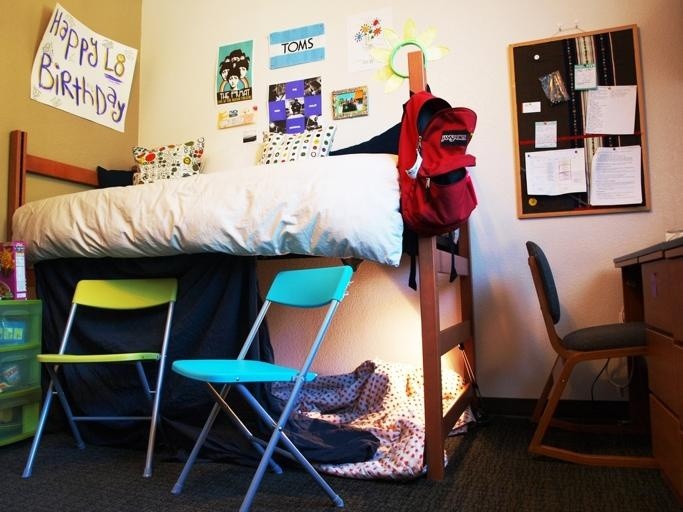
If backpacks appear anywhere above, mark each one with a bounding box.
[397,84,479,237]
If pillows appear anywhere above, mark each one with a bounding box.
[97,165,133,188]
[133,137,205,186]
[256,125,337,164]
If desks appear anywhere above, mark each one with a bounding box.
[613,236,683,511]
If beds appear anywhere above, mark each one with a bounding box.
[6,50,476,482]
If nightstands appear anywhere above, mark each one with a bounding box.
[0,299,42,447]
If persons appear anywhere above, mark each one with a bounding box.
[220,49,250,91]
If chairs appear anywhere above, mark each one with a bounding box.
[21,278,179,478]
[171,265,354,512]
[526,242,652,470]
